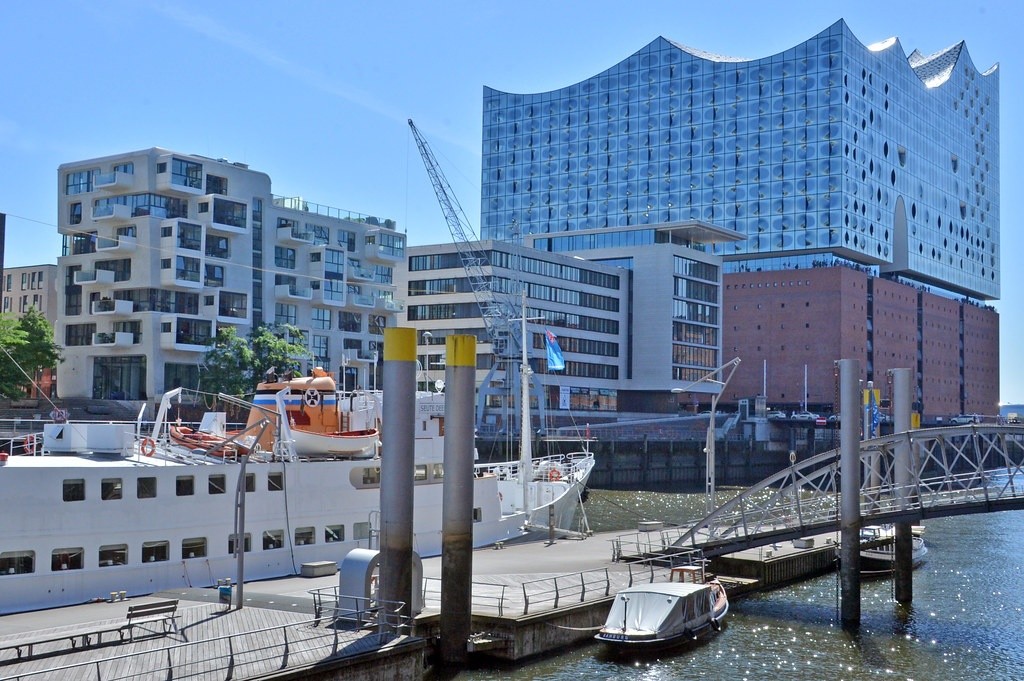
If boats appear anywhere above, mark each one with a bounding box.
[592,565,731,653]
[834,527,927,574]
[0,283,598,619]
[169,424,257,459]
[281,420,380,456]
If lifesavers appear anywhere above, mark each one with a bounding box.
[24,436,34,455]
[550,469,561,481]
[684,627,698,642]
[710,617,722,632]
[141,438,156,457]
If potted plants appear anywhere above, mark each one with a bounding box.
[97,333,108,343]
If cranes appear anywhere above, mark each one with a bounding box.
[407,115,551,437]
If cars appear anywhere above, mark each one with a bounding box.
[790,412,821,421]
[829,412,842,422]
[950,415,980,424]
[1010,419,1021,424]
[880,414,891,425]
[697,411,713,416]
[766,410,786,420]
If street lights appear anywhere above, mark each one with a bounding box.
[671,356,741,518]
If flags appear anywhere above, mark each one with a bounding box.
[545,327,566,370]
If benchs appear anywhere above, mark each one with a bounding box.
[0,599,181,659]
